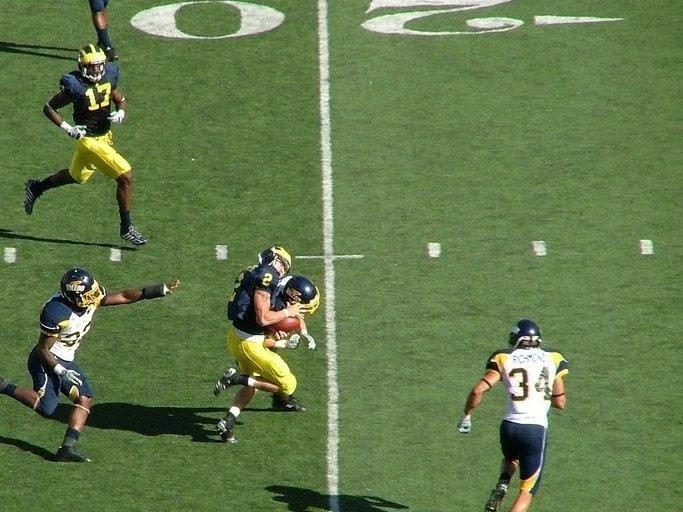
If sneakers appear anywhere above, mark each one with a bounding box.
[119,226,147,246]
[213,368,236,396]
[484,483,508,512]
[104,44,118,62]
[24,180,37,216]
[216,420,237,444]
[55,445,90,462]
[270,395,305,412]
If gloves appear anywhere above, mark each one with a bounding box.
[300,331,315,349]
[286,334,299,349]
[457,415,472,433]
[65,125,87,140]
[60,368,83,387]
[107,111,123,124]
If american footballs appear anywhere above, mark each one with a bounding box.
[265,309,300,332]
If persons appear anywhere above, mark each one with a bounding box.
[264,276,319,413]
[1,267,181,463]
[213,245,307,446]
[23,43,148,246]
[457,319,570,512]
[88,0,117,62]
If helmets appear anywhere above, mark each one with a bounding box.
[61,269,107,309]
[284,274,320,315]
[507,320,542,349]
[77,44,107,82]
[258,246,292,278]
